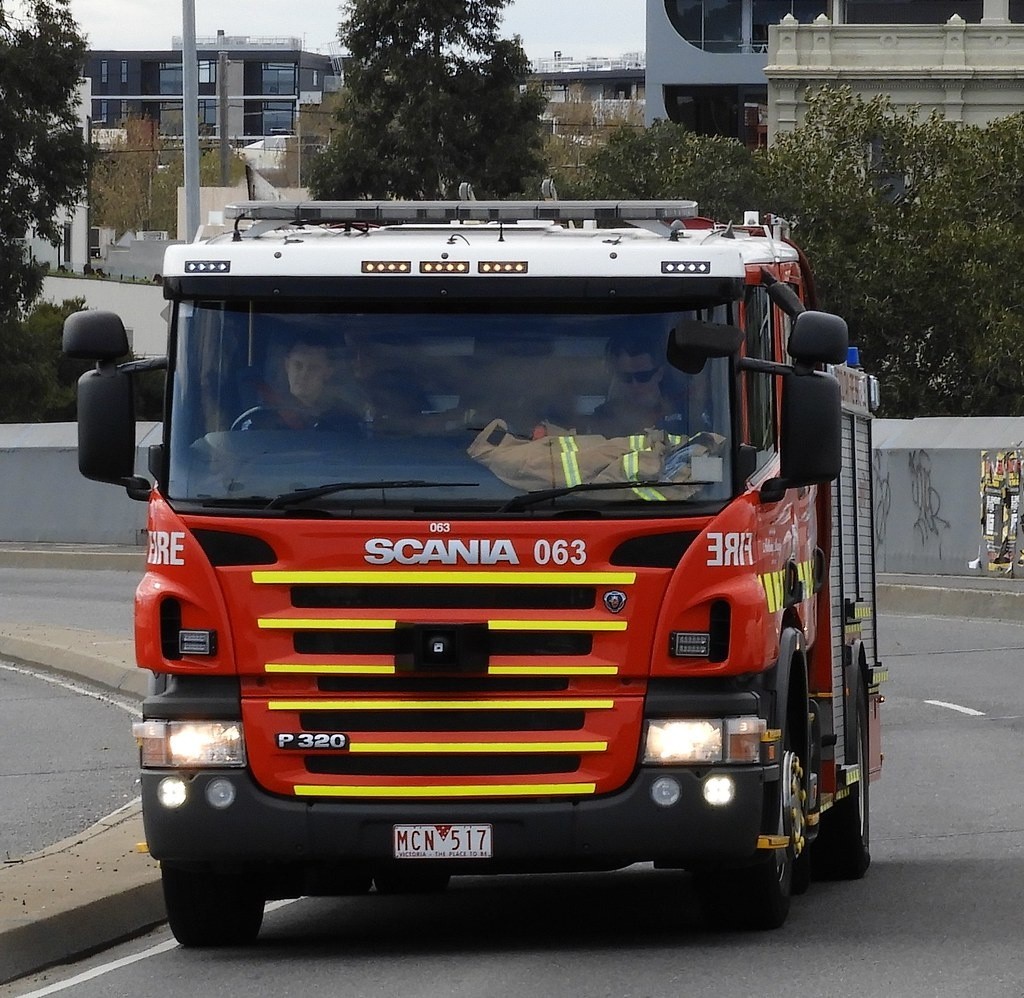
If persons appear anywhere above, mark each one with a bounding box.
[259,323,685,438]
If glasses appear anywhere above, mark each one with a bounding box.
[619,364,663,384]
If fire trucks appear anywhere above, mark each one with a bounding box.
[61,180,890,951]
